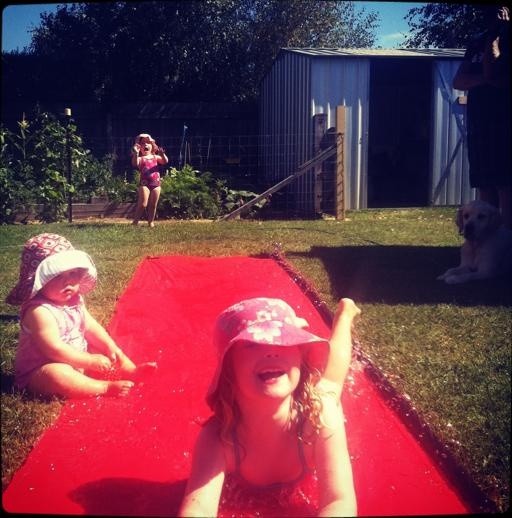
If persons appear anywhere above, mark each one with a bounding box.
[2,230,160,399]
[128,132,168,229]
[180,297,362,518]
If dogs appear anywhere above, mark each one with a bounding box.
[433,198,510,285]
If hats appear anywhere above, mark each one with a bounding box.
[204,297,330,424]
[135,133,159,155]
[6,233,97,308]
